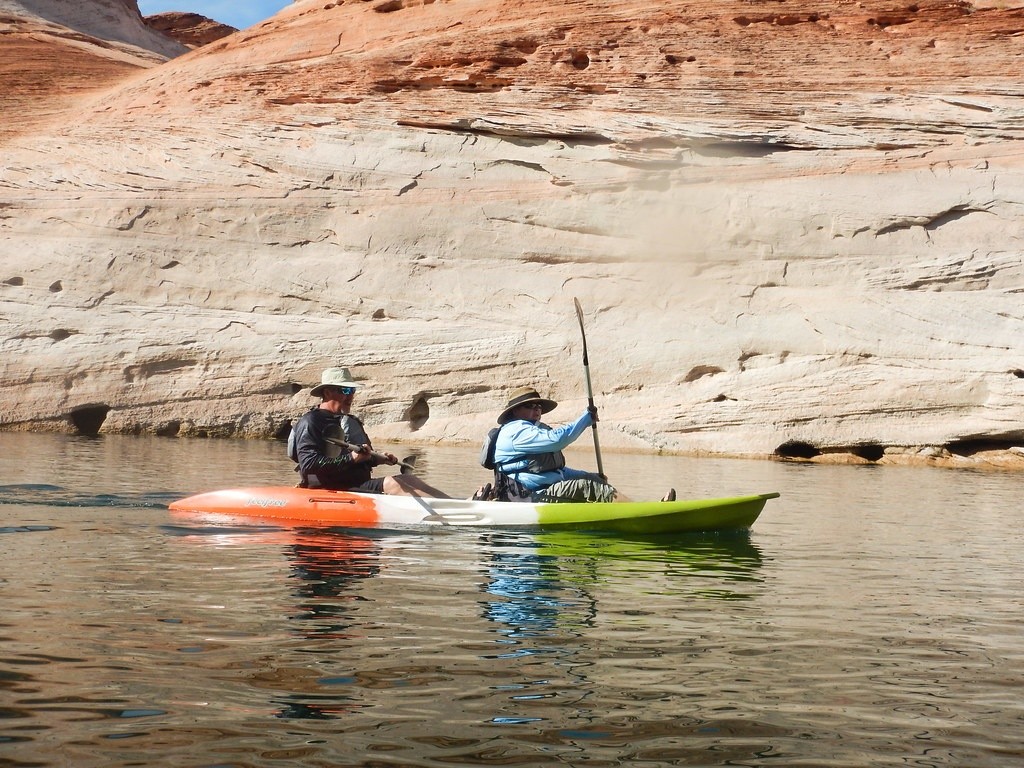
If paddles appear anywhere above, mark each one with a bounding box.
[571,296,605,474]
[326,438,416,476]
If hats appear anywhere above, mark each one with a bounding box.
[497,387,558,424]
[310,367,365,398]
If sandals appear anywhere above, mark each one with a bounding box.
[661,488,676,501]
[472,483,491,500]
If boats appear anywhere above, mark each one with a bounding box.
[169,487,779,535]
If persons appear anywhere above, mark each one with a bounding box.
[294,367,495,501]
[490,386,677,503]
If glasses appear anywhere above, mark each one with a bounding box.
[338,387,356,395]
[522,402,543,409]
